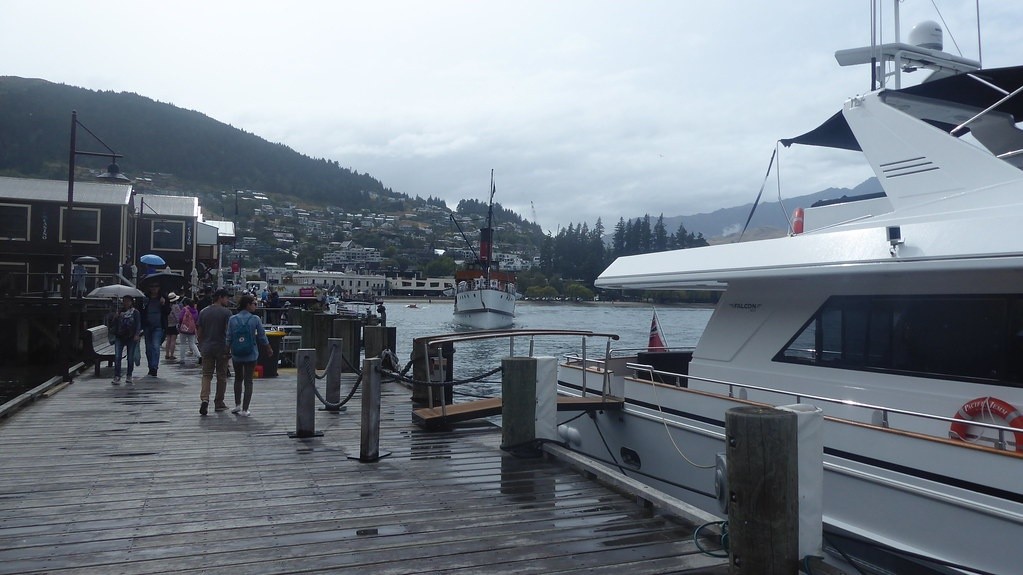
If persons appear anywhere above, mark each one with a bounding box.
[123,260,132,287]
[141,264,156,274]
[140,280,172,377]
[164,292,181,360]
[197,286,214,315]
[226,295,273,416]
[109,295,141,384]
[179,297,203,364]
[250,287,268,307]
[196,290,232,415]
[74,263,88,298]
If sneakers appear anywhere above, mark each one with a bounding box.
[111,376,120,384]
[126,375,132,383]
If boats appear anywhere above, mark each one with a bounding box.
[549,0,1023,574]
[450,167,518,331]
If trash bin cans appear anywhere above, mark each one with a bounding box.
[256,332,286,378]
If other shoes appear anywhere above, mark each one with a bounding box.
[165,355,169,360]
[177,360,185,364]
[170,356,177,360]
[200,400,208,415]
[215,404,229,410]
[197,354,202,364]
[152,368,157,376]
[242,411,250,417]
[148,367,153,375]
[231,406,241,412]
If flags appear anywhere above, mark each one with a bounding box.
[648,313,666,352]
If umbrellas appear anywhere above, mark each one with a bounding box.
[87,284,146,315]
[74,257,100,272]
[138,274,185,297]
[140,254,166,266]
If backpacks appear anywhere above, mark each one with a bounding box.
[231,315,256,357]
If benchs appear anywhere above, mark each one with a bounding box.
[85,325,128,377]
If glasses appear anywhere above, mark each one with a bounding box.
[148,285,160,288]
[249,303,257,306]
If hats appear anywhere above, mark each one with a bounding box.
[213,289,233,300]
[167,292,180,302]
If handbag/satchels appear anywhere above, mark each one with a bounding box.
[180,308,195,333]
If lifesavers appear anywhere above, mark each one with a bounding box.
[458,281,467,290]
[949,395,1022,453]
[792,207,804,233]
